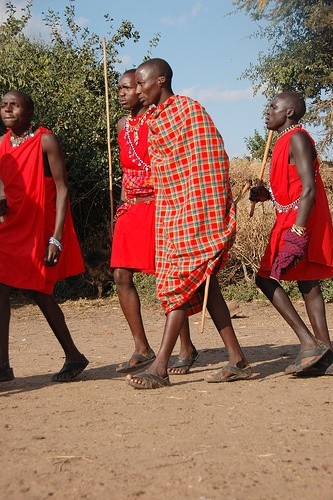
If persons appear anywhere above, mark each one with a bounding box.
[0,89,89,382]
[110,58,252,389]
[249,92,333,375]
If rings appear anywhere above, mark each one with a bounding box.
[53,259,58,262]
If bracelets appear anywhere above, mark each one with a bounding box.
[48,236,63,252]
[291,224,306,236]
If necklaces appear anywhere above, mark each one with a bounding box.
[125,103,155,145]
[10,126,34,147]
[276,124,300,137]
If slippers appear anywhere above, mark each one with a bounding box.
[292,361,333,376]
[203,364,253,383]
[0,367,15,382]
[126,369,170,389]
[169,352,201,376]
[283,343,330,375]
[51,353,89,382]
[115,353,157,373]
